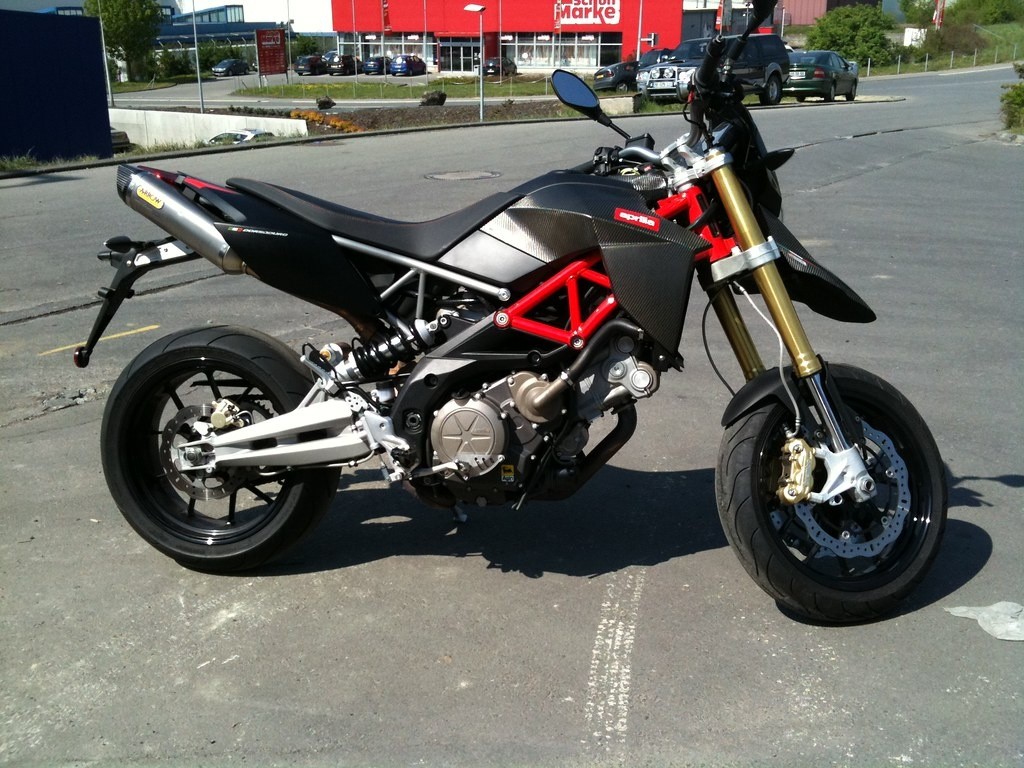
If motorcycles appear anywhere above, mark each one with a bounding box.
[70,2,950,621]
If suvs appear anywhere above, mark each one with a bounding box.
[293,56,327,76]
[646,32,790,105]
[326,54,362,76]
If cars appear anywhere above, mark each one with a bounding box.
[592,60,639,95]
[252,60,258,71]
[782,50,860,101]
[211,58,250,77]
[635,46,670,95]
[389,54,426,76]
[362,56,392,76]
[479,55,518,78]
[322,50,338,64]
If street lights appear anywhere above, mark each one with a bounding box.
[287,18,295,85]
[463,4,486,121]
